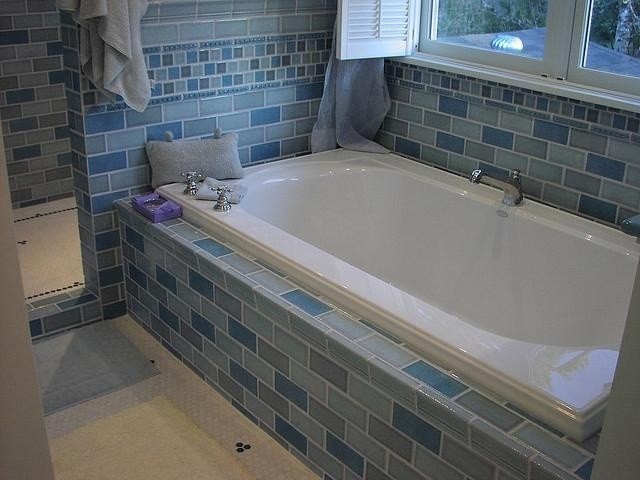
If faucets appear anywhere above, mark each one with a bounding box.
[468,168,523,208]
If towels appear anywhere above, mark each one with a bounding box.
[59,0,150,113]
[194,177,249,204]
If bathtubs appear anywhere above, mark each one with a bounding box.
[154,147,640,444]
